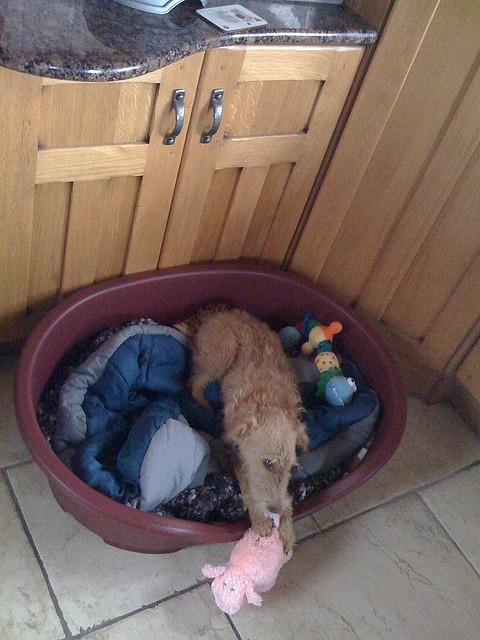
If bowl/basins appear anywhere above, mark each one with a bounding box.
[14,262,407,553]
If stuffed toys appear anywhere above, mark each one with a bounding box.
[200,512,294,614]
[281,313,358,408]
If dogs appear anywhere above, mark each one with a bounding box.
[173,302,312,556]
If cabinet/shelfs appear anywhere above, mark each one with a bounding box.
[0,45,372,344]
